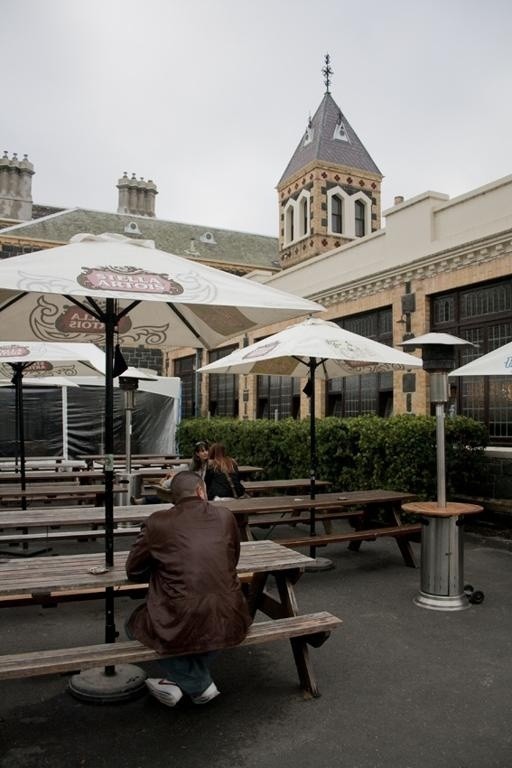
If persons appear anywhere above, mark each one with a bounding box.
[164,442,210,489]
[204,442,249,536]
[123,472,253,710]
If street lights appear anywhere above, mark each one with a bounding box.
[115,364,157,534]
[394,332,482,612]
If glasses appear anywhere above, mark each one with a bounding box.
[196,441,206,445]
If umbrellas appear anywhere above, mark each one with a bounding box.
[1,376,82,475]
[199,313,424,569]
[1,230,333,679]
[1,340,113,550]
[449,337,511,382]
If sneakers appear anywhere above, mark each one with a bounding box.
[145,678,183,707]
[191,681,219,705]
